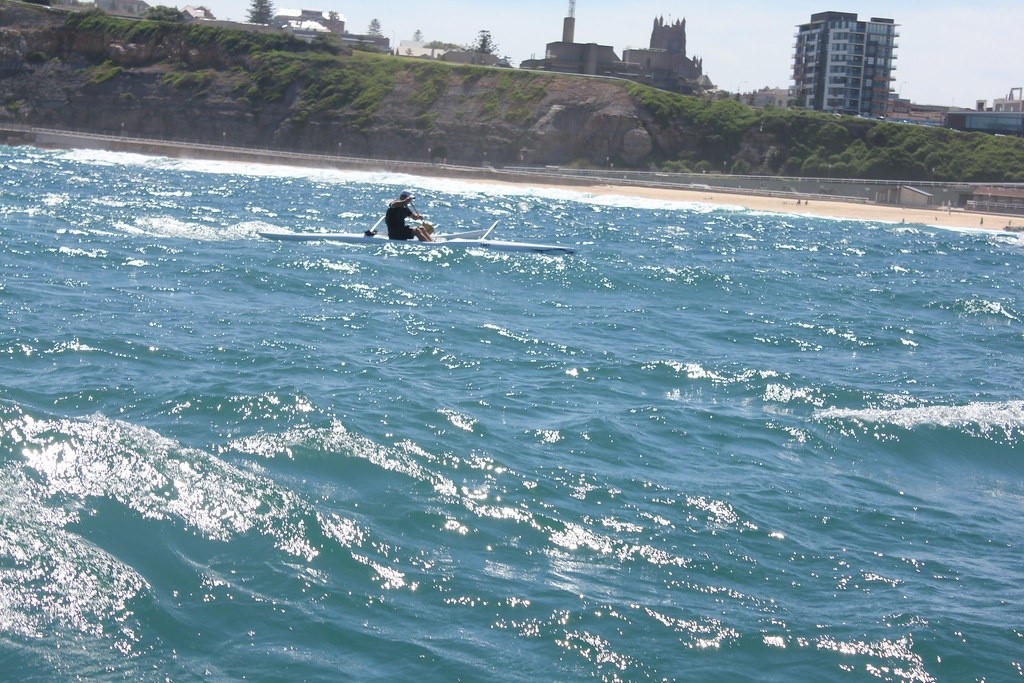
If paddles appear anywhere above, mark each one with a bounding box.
[410,201,435,235]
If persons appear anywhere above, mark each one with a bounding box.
[385,188,435,242]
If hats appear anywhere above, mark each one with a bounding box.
[401,190,416,199]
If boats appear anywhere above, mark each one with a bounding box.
[260,229,575,257]
